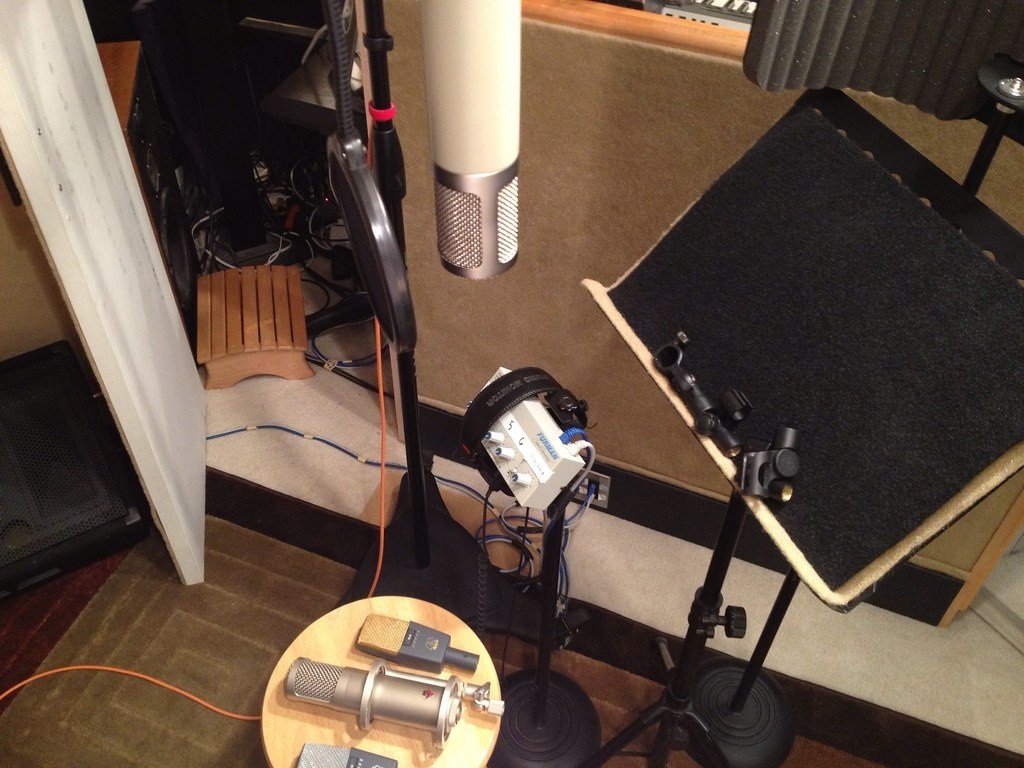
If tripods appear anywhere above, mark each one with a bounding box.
[579,86,1024,768]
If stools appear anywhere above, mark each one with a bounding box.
[260,595,503,768]
[195,264,317,391]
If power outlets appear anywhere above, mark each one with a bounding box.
[572,468,612,509]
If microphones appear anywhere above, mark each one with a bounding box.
[296,744,398,768]
[284,657,465,749]
[419,0,521,279]
[357,614,480,675]
